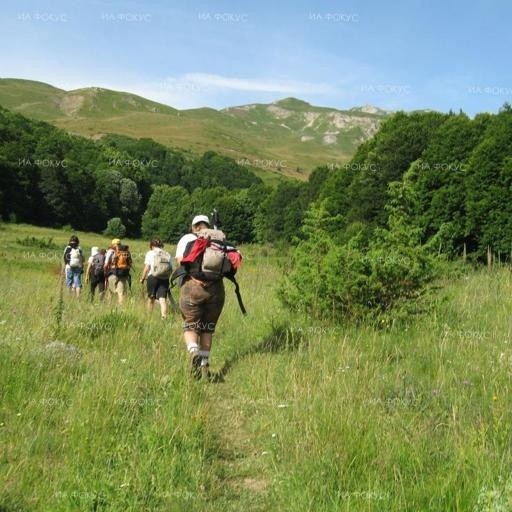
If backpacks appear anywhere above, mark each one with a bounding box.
[113,249,132,270]
[68,246,81,269]
[92,254,106,277]
[189,228,241,279]
[152,249,173,280]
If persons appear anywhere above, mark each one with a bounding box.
[172,214,226,382]
[62,232,85,296]
[84,238,133,306]
[138,236,173,319]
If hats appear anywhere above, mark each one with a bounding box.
[192,214,211,226]
[112,238,121,247]
[90,247,100,256]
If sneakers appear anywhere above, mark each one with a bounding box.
[202,365,212,379]
[189,352,203,381]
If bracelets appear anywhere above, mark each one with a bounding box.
[142,276,146,280]
